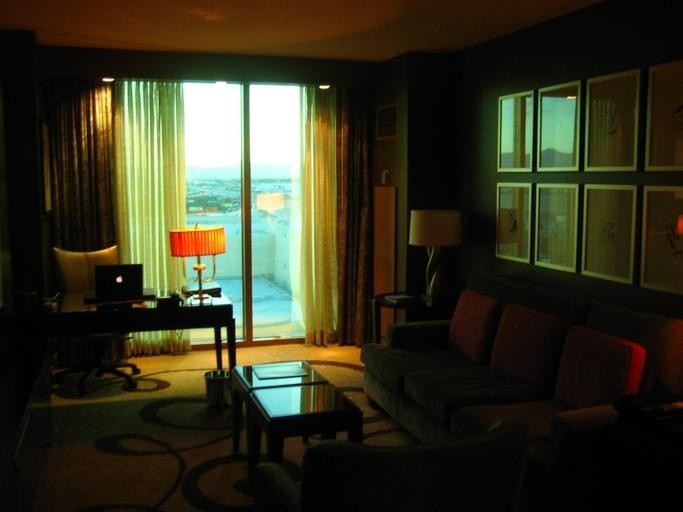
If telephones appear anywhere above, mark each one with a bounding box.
[157,292,183,308]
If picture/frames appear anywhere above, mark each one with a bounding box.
[533,183,583,274]
[374,103,400,141]
[584,67,642,173]
[644,59,683,172]
[496,88,536,174]
[580,183,638,286]
[494,181,533,264]
[639,185,683,298]
[536,78,584,173]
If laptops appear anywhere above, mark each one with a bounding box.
[95,265,142,306]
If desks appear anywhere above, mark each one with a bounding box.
[38,286,236,402]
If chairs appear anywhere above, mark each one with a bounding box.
[49,245,141,391]
[248,417,529,512]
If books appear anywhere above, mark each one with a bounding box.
[182,281,221,295]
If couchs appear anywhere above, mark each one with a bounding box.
[360,268,682,512]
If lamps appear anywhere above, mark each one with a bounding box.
[169,222,227,304]
[408,208,462,298]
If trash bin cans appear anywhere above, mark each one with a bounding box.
[205,370,232,406]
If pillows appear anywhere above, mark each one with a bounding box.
[554,324,650,409]
[491,302,566,383]
[446,288,501,367]
